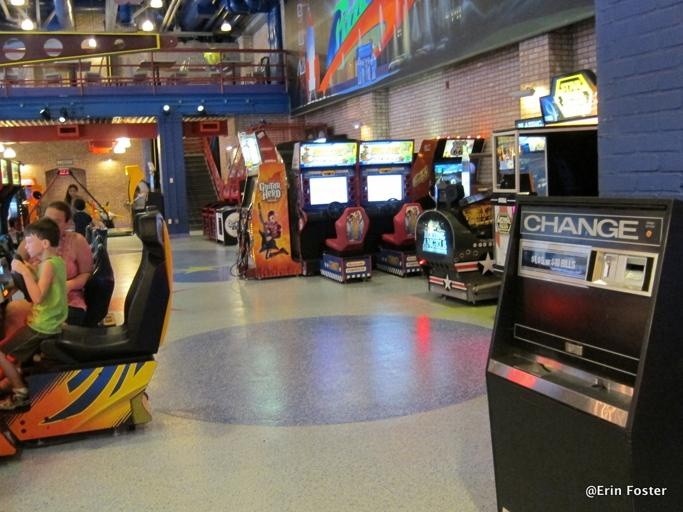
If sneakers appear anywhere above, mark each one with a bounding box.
[0,387,31,411]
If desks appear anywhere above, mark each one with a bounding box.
[0,60,253,85]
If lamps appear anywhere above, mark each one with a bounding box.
[141,10,154,32]
[113,137,131,154]
[221,19,232,32]
[197,104,207,116]
[40,108,50,121]
[58,107,68,122]
[0,142,16,159]
[162,104,171,116]
[21,0,34,31]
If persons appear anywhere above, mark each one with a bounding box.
[0,185,95,414]
[129,186,146,236]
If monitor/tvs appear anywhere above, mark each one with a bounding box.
[308,175,349,207]
[434,160,479,191]
[365,173,405,204]
[8,193,18,225]
